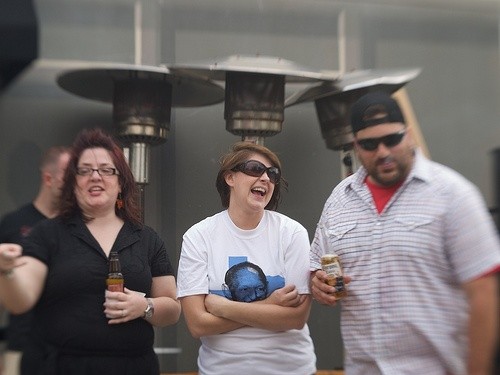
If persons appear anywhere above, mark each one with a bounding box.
[309,94,500,375]
[0,143,74,375]
[0,128,182,375]
[175,142,317,375]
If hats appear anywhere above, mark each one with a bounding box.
[350,92,405,132]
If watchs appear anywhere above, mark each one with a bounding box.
[144,298,155,324]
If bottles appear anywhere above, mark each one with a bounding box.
[106,251,123,292]
[319,226,347,299]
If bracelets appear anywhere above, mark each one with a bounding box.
[0,267,14,275]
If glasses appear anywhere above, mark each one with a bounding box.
[356,132,404,151]
[75,166,120,176]
[231,159,282,185]
